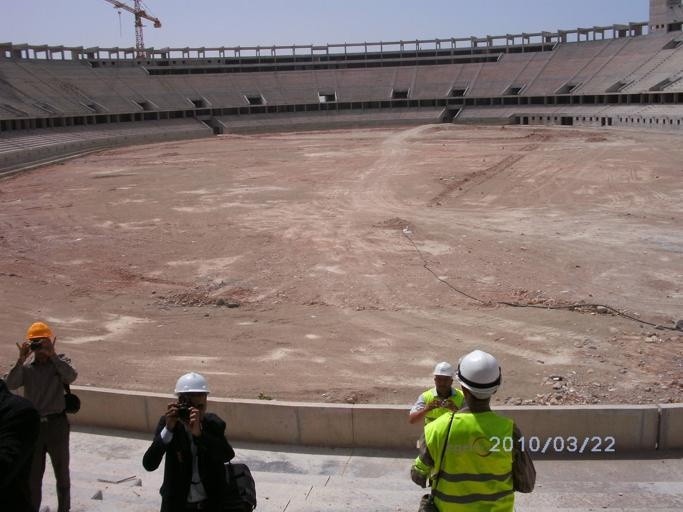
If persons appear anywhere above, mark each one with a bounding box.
[141,372,234,512]
[410,349,536,512]
[408,361,469,426]
[3,322,78,511]
[0,377,41,512]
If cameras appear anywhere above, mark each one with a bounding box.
[31,342,42,350]
[437,401,446,406]
[175,403,190,417]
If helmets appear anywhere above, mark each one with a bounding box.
[25,322,53,340]
[455,350,501,400]
[174,372,210,394]
[432,362,454,377]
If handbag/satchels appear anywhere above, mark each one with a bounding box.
[418,494,439,512]
[64,393,80,414]
[225,464,256,512]
